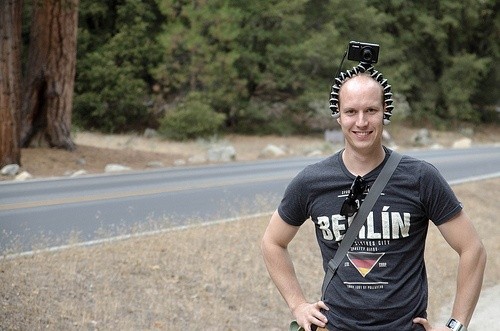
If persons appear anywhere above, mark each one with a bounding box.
[259,41,488,331]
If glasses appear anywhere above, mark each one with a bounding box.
[340,175,368,217]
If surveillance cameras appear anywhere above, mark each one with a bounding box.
[348,41,379,63]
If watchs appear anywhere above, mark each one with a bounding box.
[446,318,468,331]
